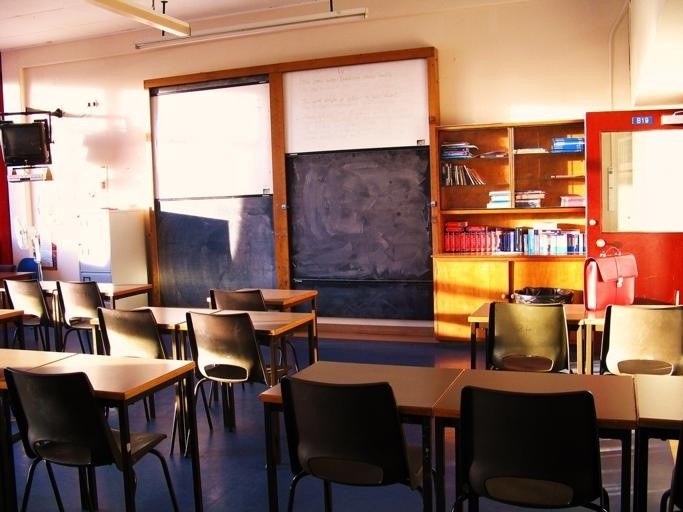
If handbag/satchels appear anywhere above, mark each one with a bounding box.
[583,246,638,313]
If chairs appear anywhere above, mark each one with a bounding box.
[182,310,288,459]
[209,287,299,374]
[0,274,106,354]
[485,301,574,375]
[452,384,610,508]
[95,306,172,420]
[279,375,437,510]
[4,368,178,510]
[599,303,683,381]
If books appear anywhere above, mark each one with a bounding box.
[439,134,587,258]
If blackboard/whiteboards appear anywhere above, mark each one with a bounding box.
[155,193,279,307]
[286,144,434,284]
[282,58,431,154]
[150,84,273,198]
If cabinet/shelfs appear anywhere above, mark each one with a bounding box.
[436,124,587,352]
[53,209,152,310]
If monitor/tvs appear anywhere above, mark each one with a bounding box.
[0,122,51,166]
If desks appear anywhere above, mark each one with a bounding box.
[437,368,635,509]
[178,310,318,388]
[27,352,203,511]
[238,287,318,321]
[632,374,683,511]
[258,360,461,508]
[92,304,215,361]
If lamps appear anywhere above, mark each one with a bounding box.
[83,1,195,42]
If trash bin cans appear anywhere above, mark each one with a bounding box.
[515,287,574,304]
